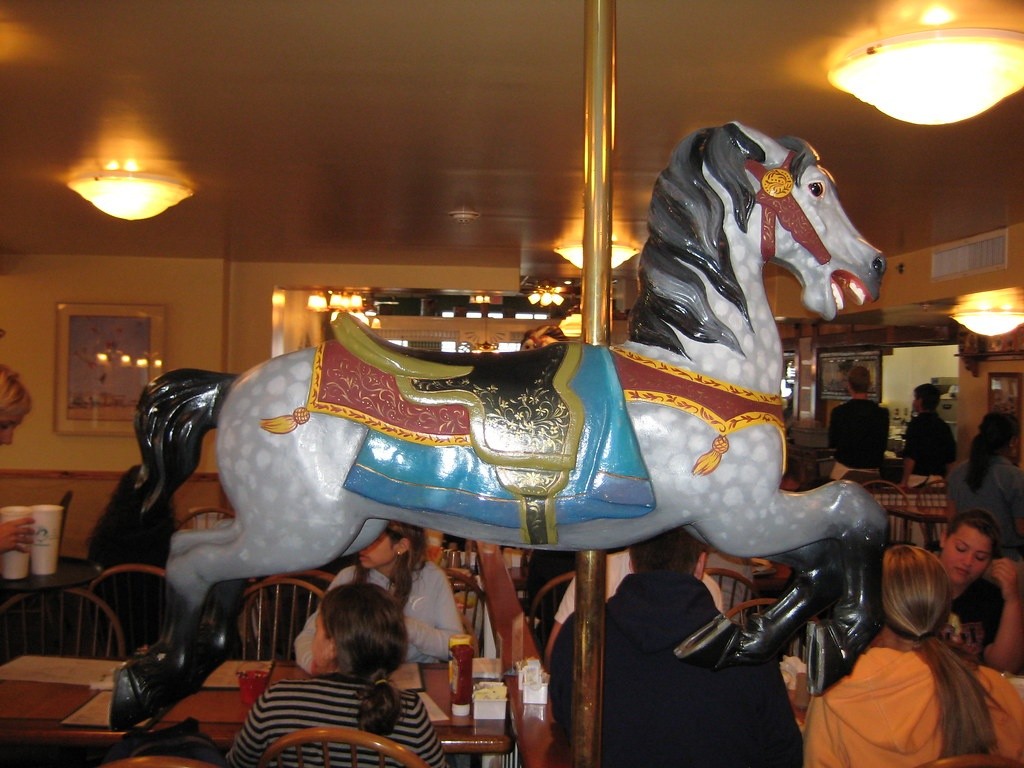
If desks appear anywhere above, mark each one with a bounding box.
[0,655,514,768]
[887,503,949,549]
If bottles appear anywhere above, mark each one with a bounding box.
[449,634,471,716]
[794,673,810,712]
[892,407,911,427]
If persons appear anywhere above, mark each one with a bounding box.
[294,518,468,676]
[0,363,33,556]
[827,366,890,491]
[545,528,805,768]
[947,410,1024,563]
[939,507,1023,673]
[226,582,450,768]
[894,384,957,491]
[546,542,724,677]
[806,545,1023,768]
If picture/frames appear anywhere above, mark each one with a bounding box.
[55,303,165,437]
[987,371,1021,464]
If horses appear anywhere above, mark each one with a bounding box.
[109,121,889,732]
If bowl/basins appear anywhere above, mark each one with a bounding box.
[474,681,507,720]
[515,656,548,704]
[472,656,503,682]
[503,548,523,568]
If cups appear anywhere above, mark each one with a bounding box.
[471,552,477,565]
[0,505,63,579]
[435,542,461,567]
[239,670,268,705]
[460,551,465,566]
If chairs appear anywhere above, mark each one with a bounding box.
[259,727,431,768]
[0,506,779,661]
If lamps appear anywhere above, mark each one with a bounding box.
[307,291,382,331]
[950,310,1024,336]
[827,28,1024,125]
[67,169,190,224]
[527,286,564,307]
[554,243,640,271]
